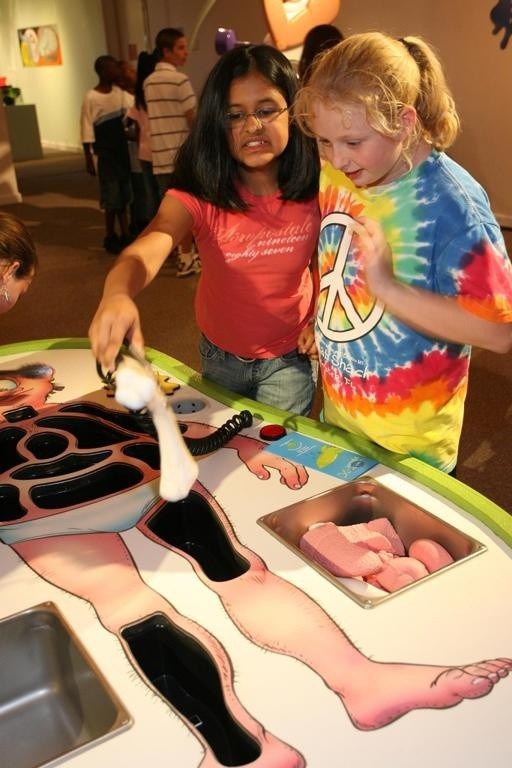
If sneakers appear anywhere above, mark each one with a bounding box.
[175,259,200,276]
[105,235,131,254]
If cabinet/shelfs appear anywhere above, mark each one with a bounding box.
[0,105,43,161]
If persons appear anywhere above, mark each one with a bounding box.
[113,61,146,235]
[123,51,157,212]
[298,30,511,479]
[298,25,344,79]
[80,56,135,255]
[1,212,39,312]
[144,27,203,278]
[87,44,321,418]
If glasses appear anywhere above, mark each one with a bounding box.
[219,104,290,128]
